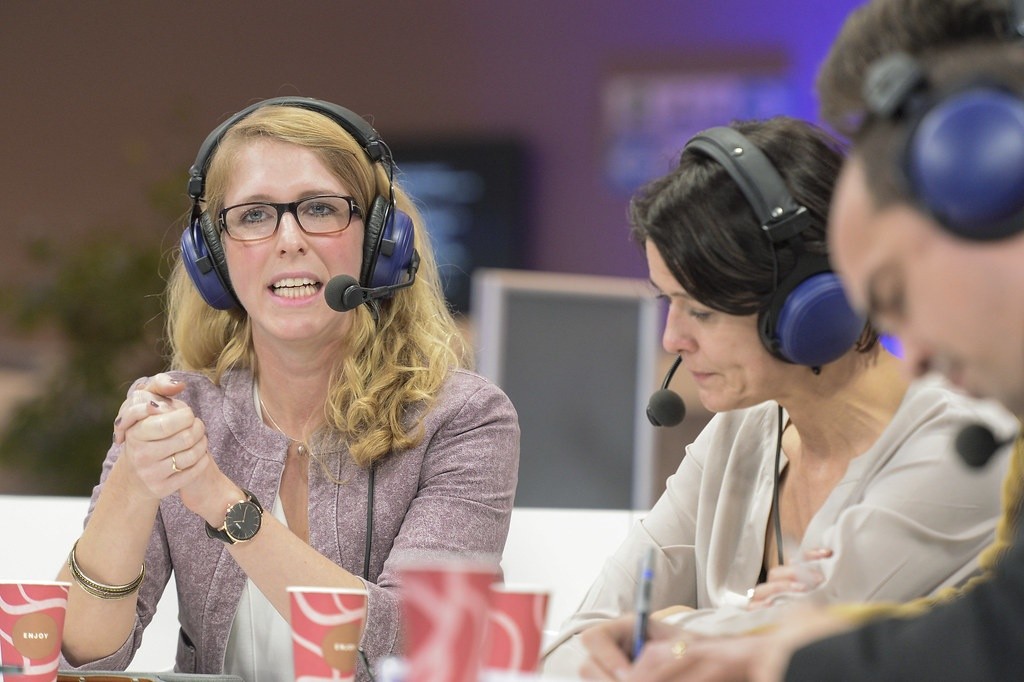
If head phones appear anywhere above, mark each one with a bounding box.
[179,95,421,317]
[681,127,867,366]
[861,53,1024,242]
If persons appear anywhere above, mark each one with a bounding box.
[576,42,1024,682]
[814,1,1024,142]
[538,117,1022,682]
[51,105,520,682]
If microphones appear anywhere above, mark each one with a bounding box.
[958,425,1017,469]
[325,249,422,312]
[646,357,685,428]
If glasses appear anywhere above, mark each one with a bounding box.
[209,191,367,244]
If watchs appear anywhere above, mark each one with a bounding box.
[204,489,264,545]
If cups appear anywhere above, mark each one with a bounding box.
[0,579,73,682]
[488,583,553,677]
[281,581,368,682]
[391,550,503,682]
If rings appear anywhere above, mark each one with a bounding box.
[172,453,182,473]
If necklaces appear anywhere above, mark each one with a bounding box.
[259,393,308,456]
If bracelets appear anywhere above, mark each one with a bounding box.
[67,537,145,600]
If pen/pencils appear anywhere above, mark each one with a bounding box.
[630,547,655,660]
